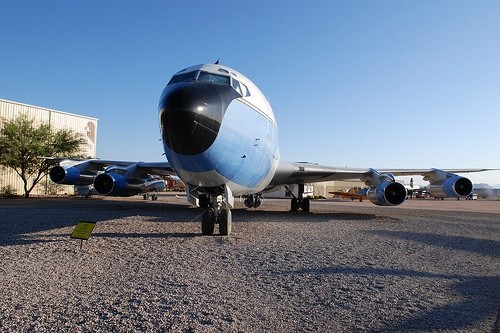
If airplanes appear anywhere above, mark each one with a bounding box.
[404,178,421,200]
[328,186,368,202]
[37,60,500,235]
